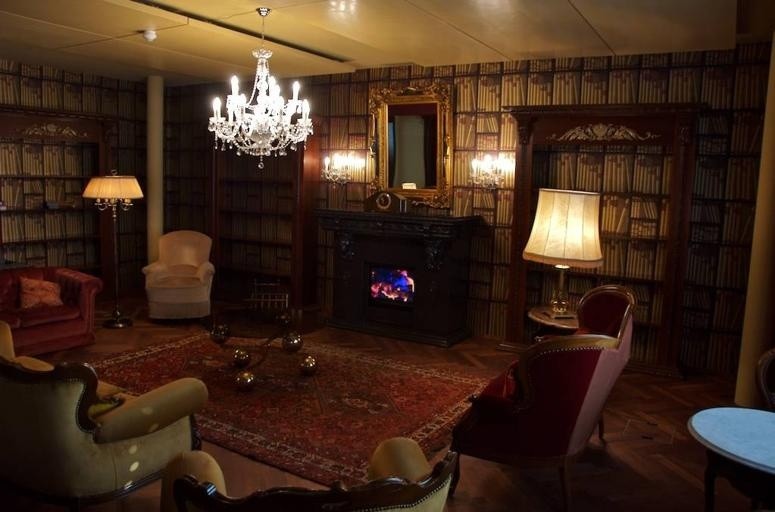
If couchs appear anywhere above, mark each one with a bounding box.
[142,230,215,320]
[0,266,104,357]
[0,319,209,512]
[160,437,461,512]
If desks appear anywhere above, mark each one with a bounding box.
[528,305,605,440]
[687,406,775,512]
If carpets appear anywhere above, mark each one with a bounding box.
[79,325,493,488]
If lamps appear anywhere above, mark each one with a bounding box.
[468,151,516,193]
[321,151,368,191]
[522,187,606,321]
[82,169,145,330]
[208,7,314,169]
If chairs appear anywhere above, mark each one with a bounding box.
[572,284,638,467]
[508,333,619,512]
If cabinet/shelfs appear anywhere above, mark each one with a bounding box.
[501,102,694,367]
[164,41,773,379]
[1,58,148,302]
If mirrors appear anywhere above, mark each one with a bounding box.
[366,82,453,210]
